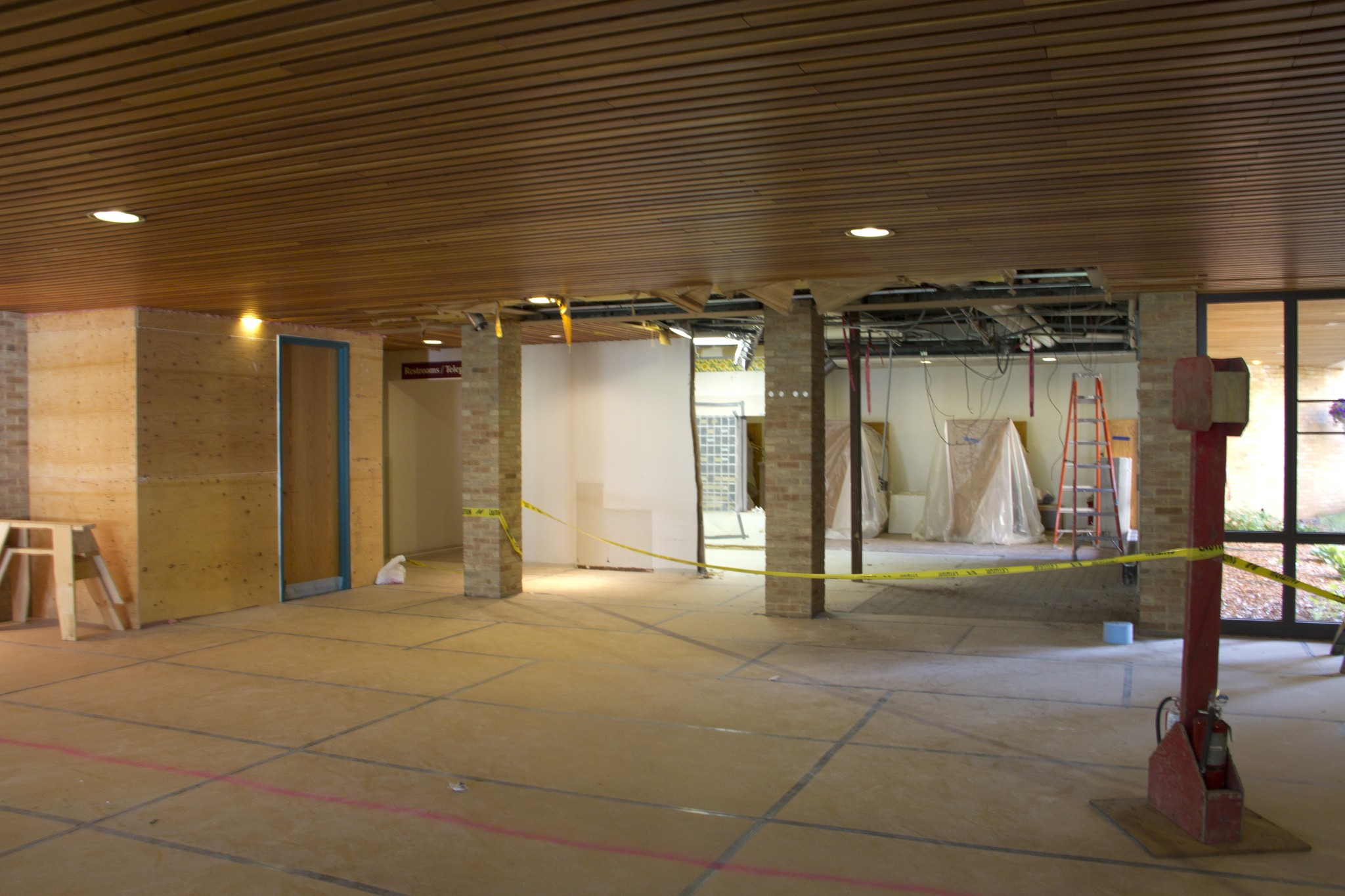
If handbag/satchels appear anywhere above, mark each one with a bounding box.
[376,555,406,584]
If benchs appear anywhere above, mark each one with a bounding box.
[0,519,124,641]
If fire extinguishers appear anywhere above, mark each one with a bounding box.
[1155,694,1181,745]
[1190,690,1231,789]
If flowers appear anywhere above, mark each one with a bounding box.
[1328,398,1345,426]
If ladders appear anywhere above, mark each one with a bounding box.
[1052,374,1127,556]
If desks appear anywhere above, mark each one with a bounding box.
[1038,504,1065,530]
